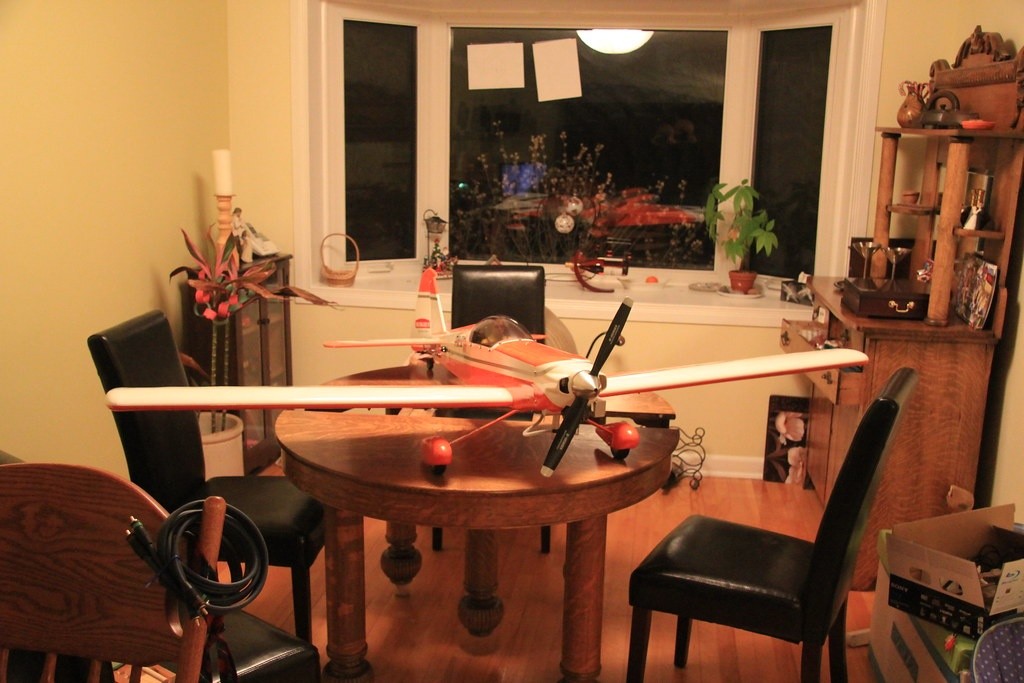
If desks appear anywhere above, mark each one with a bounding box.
[276,367,680,683]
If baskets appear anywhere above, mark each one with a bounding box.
[319,233,359,287]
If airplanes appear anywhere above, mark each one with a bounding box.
[102,264,868,483]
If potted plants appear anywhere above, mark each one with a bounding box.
[167,224,336,483]
[707,179,778,293]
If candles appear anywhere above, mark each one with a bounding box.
[211,147,232,196]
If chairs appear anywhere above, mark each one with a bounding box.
[625,368,918,683]
[431,265,551,552]
[0,310,326,683]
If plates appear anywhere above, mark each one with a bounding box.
[834,280,845,290]
[961,120,996,130]
[689,283,721,291]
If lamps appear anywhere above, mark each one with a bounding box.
[578,28,654,54]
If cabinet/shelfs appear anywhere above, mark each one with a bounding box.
[781,276,997,589]
[185,254,293,472]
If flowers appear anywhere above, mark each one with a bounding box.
[765,409,808,486]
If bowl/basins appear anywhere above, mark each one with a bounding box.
[902,191,919,205]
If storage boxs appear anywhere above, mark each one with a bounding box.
[864,504,1024,683]
